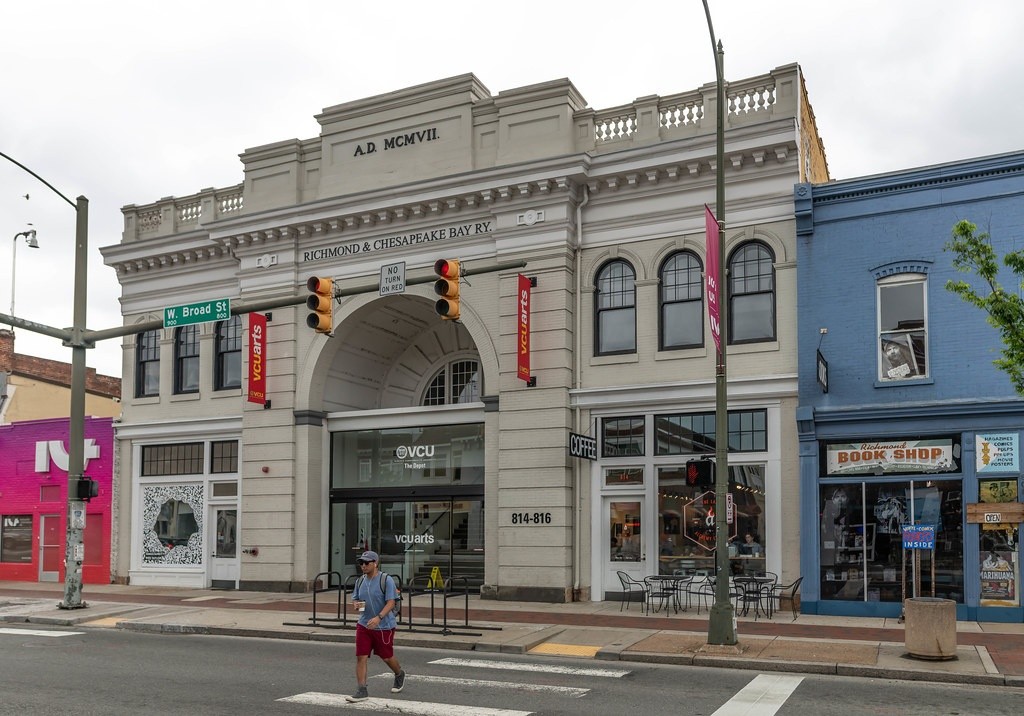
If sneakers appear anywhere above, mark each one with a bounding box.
[391,669,406,692]
[346,690,369,702]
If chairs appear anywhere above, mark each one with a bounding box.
[617,571,804,617]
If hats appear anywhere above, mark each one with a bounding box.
[358,551,379,561]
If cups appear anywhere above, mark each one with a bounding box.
[755,552,759,557]
[358,602,365,611]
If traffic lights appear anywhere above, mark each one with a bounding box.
[685,460,716,487]
[306,276,333,333]
[78,480,98,498]
[434,257,461,320]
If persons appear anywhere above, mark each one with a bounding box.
[743,533,765,557]
[883,340,914,377]
[345,551,406,702]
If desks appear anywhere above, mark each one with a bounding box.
[734,578,774,617]
[658,555,766,578]
[650,576,689,615]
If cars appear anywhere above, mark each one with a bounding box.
[159,536,188,550]
[979,543,1015,562]
[3,532,20,549]
[370,529,406,555]
[920,550,964,590]
[616,523,641,556]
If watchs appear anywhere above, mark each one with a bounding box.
[378,613,383,620]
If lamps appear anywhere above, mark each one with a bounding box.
[658,488,693,502]
[735,481,765,497]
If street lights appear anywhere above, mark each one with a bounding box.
[10,229,39,334]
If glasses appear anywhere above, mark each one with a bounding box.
[359,560,375,565]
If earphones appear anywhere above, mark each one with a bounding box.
[374,564,376,567]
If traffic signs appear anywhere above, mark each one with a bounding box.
[163,297,231,328]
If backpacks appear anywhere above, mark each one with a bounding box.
[356,572,402,614]
